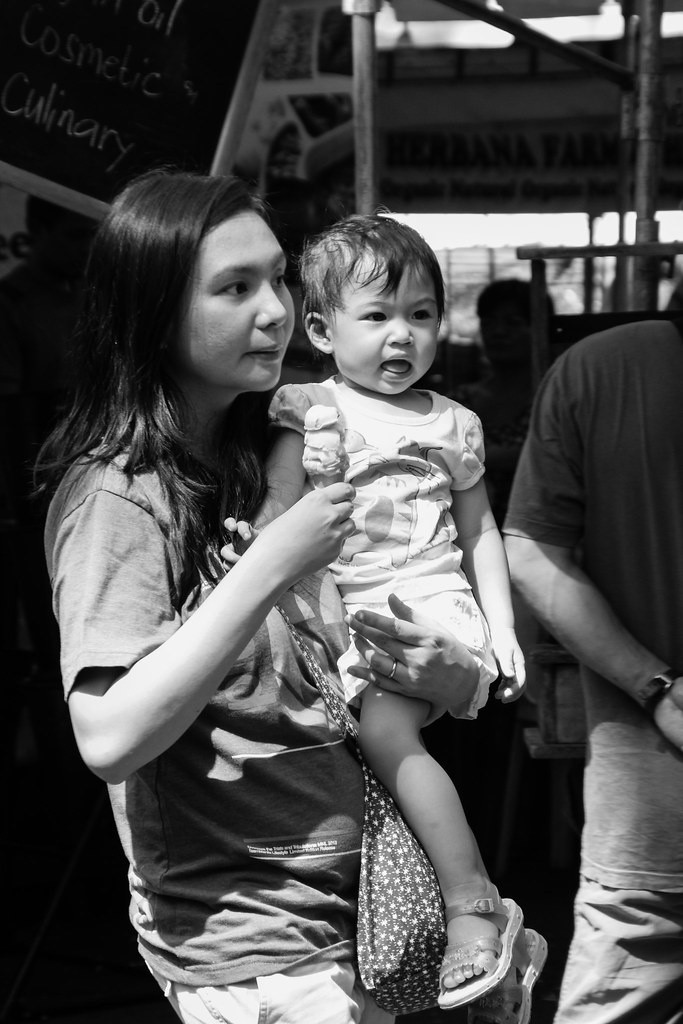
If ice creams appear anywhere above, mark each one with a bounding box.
[301,404,348,490]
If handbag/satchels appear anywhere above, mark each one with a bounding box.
[353,749,448,1017]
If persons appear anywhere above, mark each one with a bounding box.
[443,279,568,517]
[502,279,683,1023]
[222,211,547,1024]
[0,177,98,767]
[30,172,479,1024]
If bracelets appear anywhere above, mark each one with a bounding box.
[637,666,683,717]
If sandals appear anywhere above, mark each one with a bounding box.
[437,879,524,1011]
[467,928,548,1024]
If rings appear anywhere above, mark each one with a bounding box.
[387,657,397,678]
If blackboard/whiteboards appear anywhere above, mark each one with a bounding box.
[0,0,279,221]
